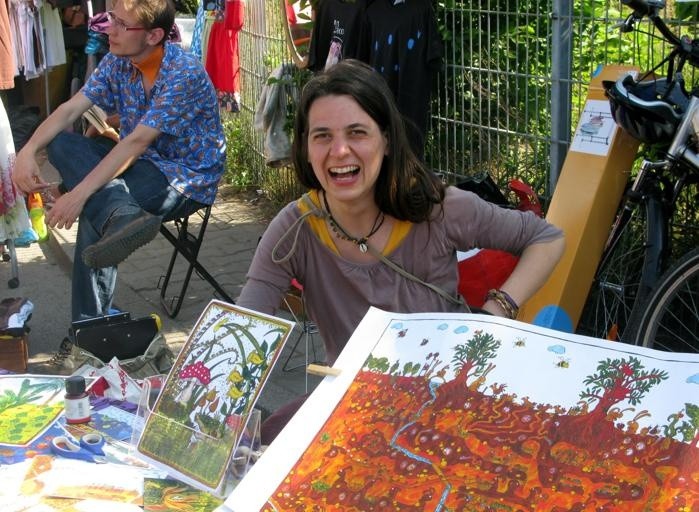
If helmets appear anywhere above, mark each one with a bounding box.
[601,76,697,143]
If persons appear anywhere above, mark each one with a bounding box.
[13,0,227,376]
[234,58,565,478]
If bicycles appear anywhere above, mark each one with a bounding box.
[580,1,699,356]
[629,245,699,354]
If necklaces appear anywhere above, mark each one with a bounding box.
[320,189,387,253]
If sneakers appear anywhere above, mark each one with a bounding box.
[82,206,161,268]
[27,337,94,376]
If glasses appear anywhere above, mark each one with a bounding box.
[105,10,146,31]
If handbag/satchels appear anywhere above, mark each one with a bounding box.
[62,315,175,377]
[458,202,543,308]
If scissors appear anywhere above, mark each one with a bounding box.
[50,432,109,465]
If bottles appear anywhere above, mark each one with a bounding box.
[63,375,91,424]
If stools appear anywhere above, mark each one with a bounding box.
[278,289,325,374]
[155,194,237,321]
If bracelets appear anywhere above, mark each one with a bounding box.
[486,285,519,320]
